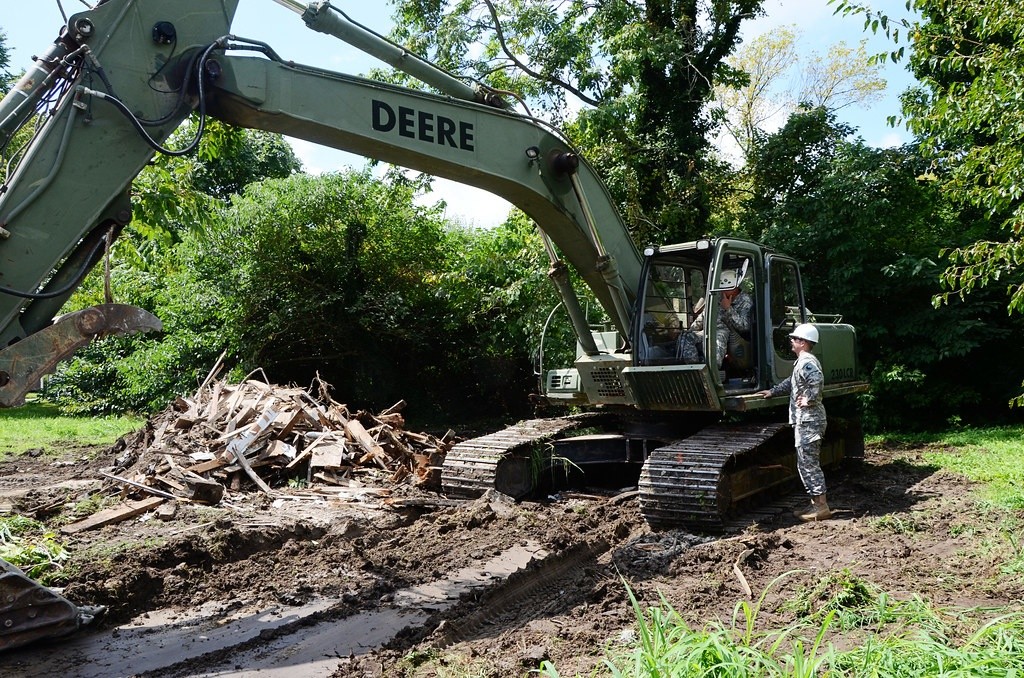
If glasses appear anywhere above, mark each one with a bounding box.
[789,336,801,341]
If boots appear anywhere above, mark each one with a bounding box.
[794,493,830,520]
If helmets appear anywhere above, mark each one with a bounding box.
[789,323,819,345]
[719,270,739,289]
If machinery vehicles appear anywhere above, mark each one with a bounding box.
[0,0,865,535]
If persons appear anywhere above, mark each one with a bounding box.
[758,324,831,521]
[681,271,753,364]
